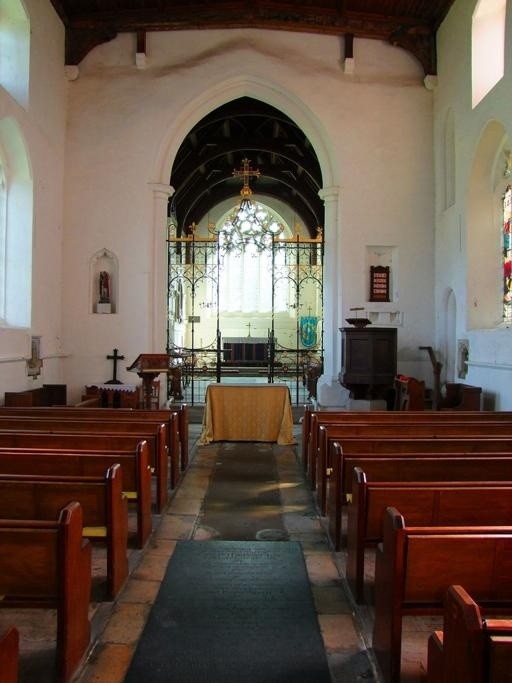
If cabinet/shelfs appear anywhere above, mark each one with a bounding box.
[338,326,399,402]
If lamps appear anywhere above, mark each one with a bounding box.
[206,155,285,259]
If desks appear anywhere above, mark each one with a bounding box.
[221,334,278,363]
